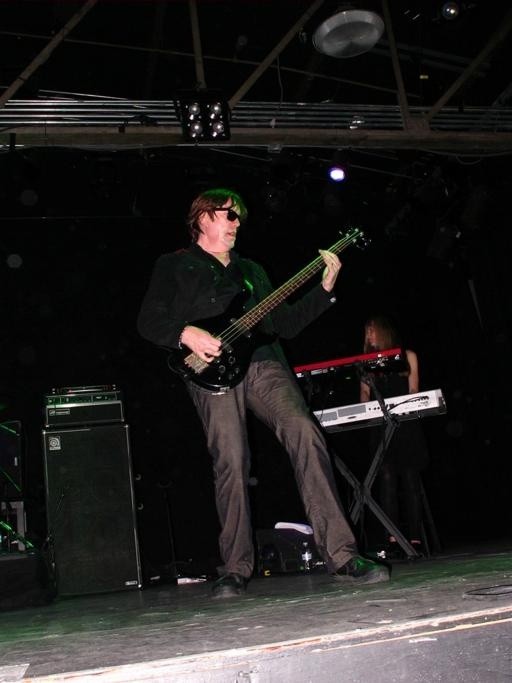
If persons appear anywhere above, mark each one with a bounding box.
[135,187,393,599]
[357,316,431,546]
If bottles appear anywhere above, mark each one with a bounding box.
[298,542,314,570]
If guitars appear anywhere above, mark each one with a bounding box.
[167,226,375,396]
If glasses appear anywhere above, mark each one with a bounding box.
[215,207,241,221]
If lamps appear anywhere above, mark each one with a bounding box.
[180,96,231,142]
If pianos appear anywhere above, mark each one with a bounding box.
[313,387,447,434]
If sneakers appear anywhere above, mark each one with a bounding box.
[332,557,388,586]
[212,573,251,598]
